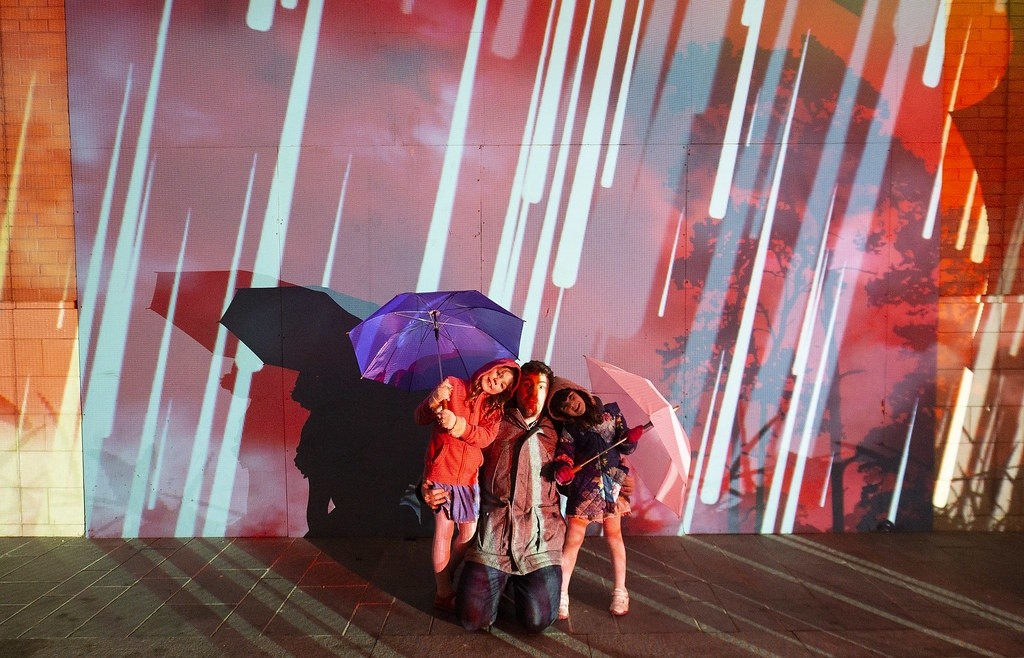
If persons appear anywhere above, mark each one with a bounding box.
[413,357,521,613]
[545,375,644,620]
[420,359,634,633]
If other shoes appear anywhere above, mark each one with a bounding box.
[433,592,458,613]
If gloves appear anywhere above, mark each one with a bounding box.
[555,466,576,485]
[623,426,642,444]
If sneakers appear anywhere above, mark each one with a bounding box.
[557,592,570,619]
[608,588,630,615]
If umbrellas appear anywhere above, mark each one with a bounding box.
[572,354,692,519]
[347,289,526,410]
